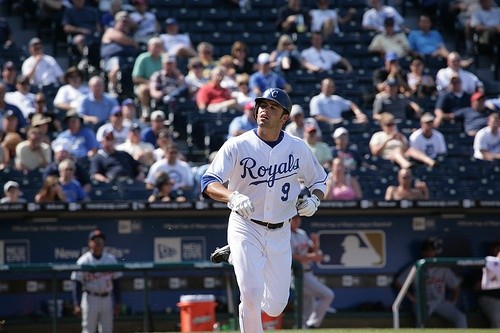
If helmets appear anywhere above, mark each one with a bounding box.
[254,87,292,131]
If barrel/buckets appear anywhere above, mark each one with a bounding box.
[177,301,218,333]
[261,310,284,330]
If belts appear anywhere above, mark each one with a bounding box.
[236,211,291,229]
[85,290,108,297]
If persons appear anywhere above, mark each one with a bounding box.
[0,0,500,203]
[291,215,334,328]
[202,87,328,333]
[398,241,467,328]
[71,230,123,333]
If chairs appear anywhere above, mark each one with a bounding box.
[0,0,499,202]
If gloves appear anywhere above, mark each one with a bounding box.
[228,191,255,217]
[297,193,320,217]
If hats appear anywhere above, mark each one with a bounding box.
[165,17,179,26]
[151,110,165,122]
[111,106,124,115]
[472,92,484,100]
[385,50,398,61]
[122,99,135,108]
[31,114,51,127]
[89,230,106,240]
[4,181,19,192]
[64,67,83,82]
[386,77,397,86]
[290,104,302,118]
[332,127,348,140]
[259,52,272,63]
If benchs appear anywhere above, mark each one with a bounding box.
[0,288,484,333]
